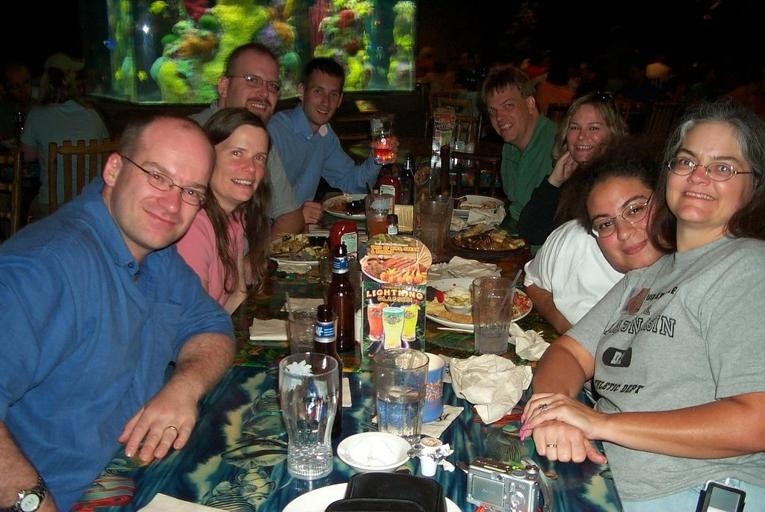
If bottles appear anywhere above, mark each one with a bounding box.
[402,152,415,203]
[313,240,356,436]
[385,212,399,236]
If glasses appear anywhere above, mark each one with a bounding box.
[227,74,280,93]
[117,151,208,206]
[592,191,653,238]
[666,158,752,181]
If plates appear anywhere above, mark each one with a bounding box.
[454,233,527,254]
[323,194,368,220]
[280,482,464,512]
[425,278,534,333]
[454,193,505,214]
[269,234,327,266]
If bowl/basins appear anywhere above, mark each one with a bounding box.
[337,432,415,474]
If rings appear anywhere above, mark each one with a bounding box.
[547,444,558,449]
[165,425,179,432]
[538,405,548,413]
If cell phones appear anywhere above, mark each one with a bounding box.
[696,479,746,512]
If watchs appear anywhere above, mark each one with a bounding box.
[0,472,49,512]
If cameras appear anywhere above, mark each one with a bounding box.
[466,456,540,512]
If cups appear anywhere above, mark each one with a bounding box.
[366,303,419,350]
[285,308,338,359]
[472,274,529,350]
[372,349,446,445]
[419,194,451,259]
[276,352,343,480]
[364,194,392,237]
[371,133,395,162]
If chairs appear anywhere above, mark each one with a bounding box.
[1,144,24,243]
[47,134,124,214]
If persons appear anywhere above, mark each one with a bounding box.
[524,216,627,335]
[0,44,665,287]
[519,96,764,511]
[1,115,237,512]
[173,107,271,318]
